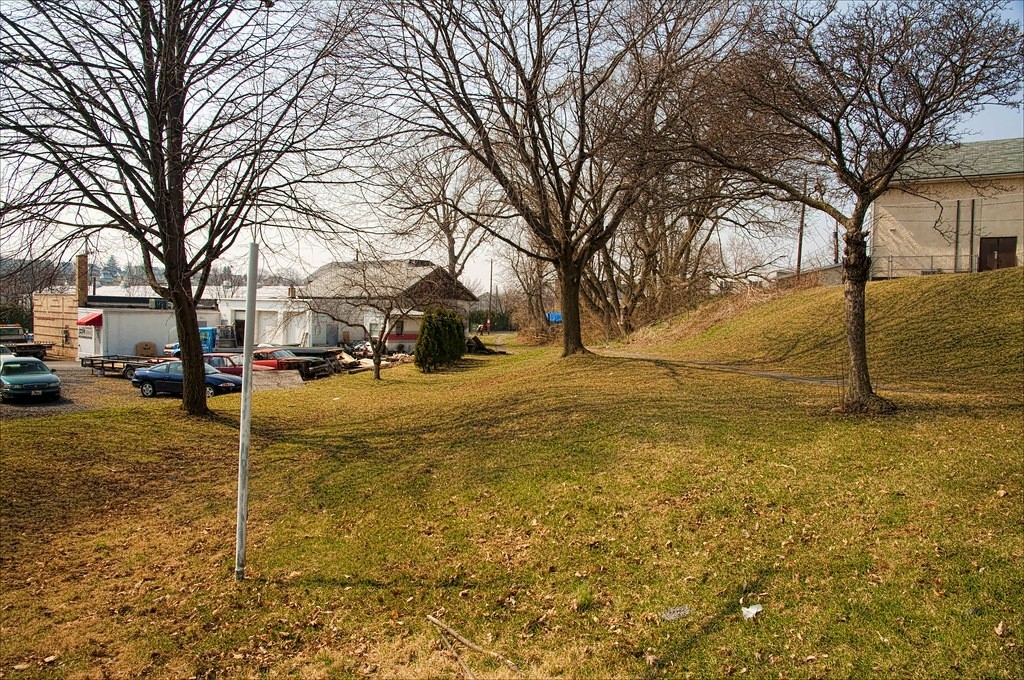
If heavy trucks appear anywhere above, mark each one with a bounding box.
[164,326,360,369]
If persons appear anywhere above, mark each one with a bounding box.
[479,326,484,336]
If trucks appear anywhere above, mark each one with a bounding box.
[0,323,56,359]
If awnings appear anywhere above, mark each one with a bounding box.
[76,313,103,326]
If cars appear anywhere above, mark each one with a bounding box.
[241,348,340,382]
[0,357,62,402]
[0,345,17,364]
[202,353,275,378]
[132,360,242,398]
